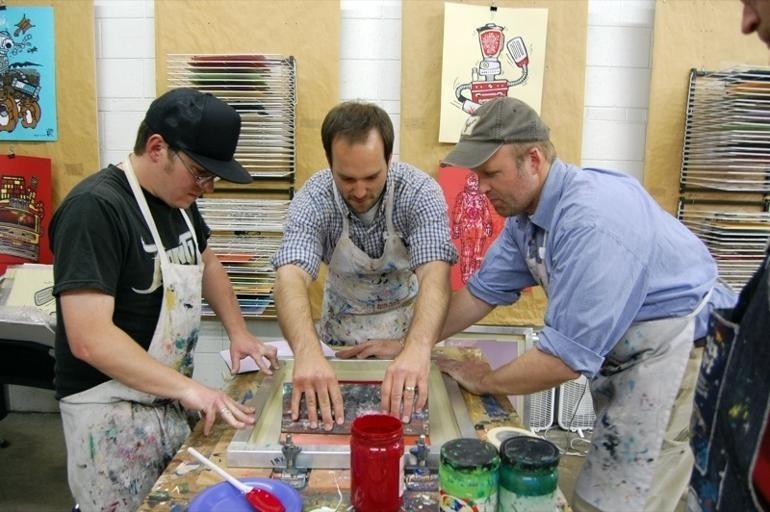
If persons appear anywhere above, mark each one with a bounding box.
[334,96,738,512]
[269,99,459,431]
[48,88,280,512]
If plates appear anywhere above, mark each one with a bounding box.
[187,475,303,510]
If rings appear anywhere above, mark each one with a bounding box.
[404,386,416,391]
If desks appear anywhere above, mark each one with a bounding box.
[135,364,576,512]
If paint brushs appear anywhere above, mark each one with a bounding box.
[188,448,287,512]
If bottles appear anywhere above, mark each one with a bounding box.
[349,414,405,512]
[438,437,500,511]
[500,436,560,511]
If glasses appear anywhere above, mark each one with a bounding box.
[173,150,225,187]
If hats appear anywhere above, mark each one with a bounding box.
[143,86,256,187]
[439,91,551,172]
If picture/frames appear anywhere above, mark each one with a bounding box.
[221,352,484,470]
[436,321,535,433]
